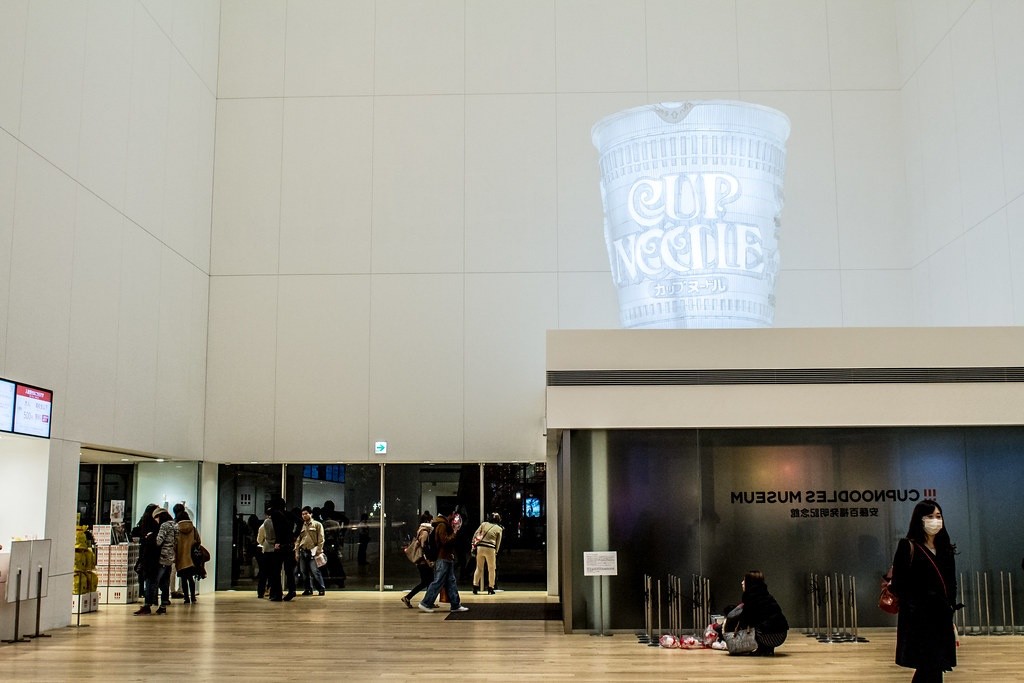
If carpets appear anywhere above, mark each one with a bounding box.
[444,602,563,621]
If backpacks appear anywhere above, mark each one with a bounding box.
[424,522,447,562]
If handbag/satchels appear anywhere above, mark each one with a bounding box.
[470,545,477,557]
[404,529,429,563]
[879,539,914,614]
[722,619,758,655]
[191,527,211,567]
[952,622,959,647]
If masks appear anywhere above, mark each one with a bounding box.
[922,519,943,535]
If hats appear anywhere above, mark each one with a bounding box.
[152,507,167,518]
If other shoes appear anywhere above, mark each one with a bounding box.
[184,601,190,605]
[258,590,264,598]
[283,592,296,601]
[269,597,282,601]
[302,590,313,595]
[152,601,171,606]
[191,599,197,604]
[752,644,775,656]
[156,605,166,614]
[319,591,324,595]
[134,606,151,616]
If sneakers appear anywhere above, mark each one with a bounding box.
[431,603,440,608]
[451,606,469,612]
[418,604,434,612]
[401,597,412,608]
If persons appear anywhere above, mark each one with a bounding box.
[230,499,351,591]
[356,513,370,566]
[890,499,957,683]
[293,506,325,596]
[401,510,440,609]
[417,502,469,613]
[470,512,503,595]
[133,508,179,616]
[268,497,304,602]
[131,504,210,604]
[713,568,789,656]
[256,507,280,598]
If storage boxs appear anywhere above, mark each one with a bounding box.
[71,525,140,614]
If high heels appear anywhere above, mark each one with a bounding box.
[487,586,495,594]
[472,585,479,594]
